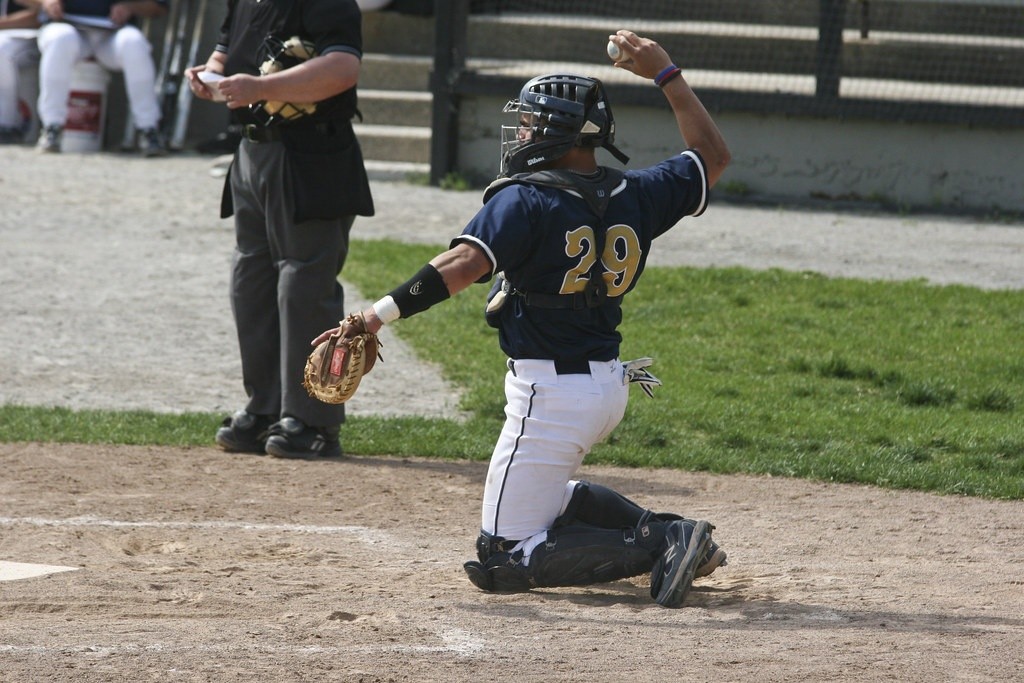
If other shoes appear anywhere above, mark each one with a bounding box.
[134,127,165,158]
[36,125,63,153]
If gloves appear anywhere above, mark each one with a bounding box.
[621,357,662,399]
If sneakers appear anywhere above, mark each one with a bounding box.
[216,420,267,451]
[695,541,727,578]
[657,519,712,609]
[265,426,343,459]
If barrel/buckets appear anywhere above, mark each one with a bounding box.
[14,58,41,142]
[53,57,110,152]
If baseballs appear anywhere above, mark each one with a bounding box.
[606,40,631,63]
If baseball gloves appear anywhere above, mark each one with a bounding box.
[300,309,386,405]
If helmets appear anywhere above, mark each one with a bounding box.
[498,74,614,176]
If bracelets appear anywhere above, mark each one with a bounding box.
[655,65,681,87]
[373,295,400,324]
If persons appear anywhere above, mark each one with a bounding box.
[0,0,163,157]
[309,28,731,610]
[184,0,375,459]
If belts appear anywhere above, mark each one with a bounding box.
[241,125,281,143]
[553,360,591,375]
